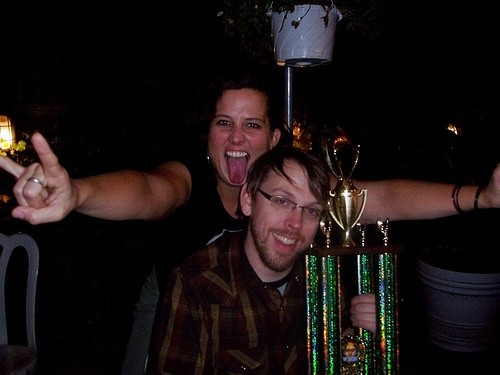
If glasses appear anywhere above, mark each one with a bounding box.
[257,187,328,223]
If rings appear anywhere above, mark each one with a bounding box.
[27,177,44,187]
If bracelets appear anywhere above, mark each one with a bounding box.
[474,186,483,209]
[452,184,465,213]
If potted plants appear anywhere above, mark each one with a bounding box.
[214,0,372,70]
[417,230,500,353]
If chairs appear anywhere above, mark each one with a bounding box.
[0,231,40,375]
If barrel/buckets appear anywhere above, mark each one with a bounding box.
[272,0,339,66]
[417,257,500,351]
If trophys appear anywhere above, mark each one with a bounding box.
[304,138,402,375]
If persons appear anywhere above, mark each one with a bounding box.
[121,145,378,375]
[0,78,500,375]
